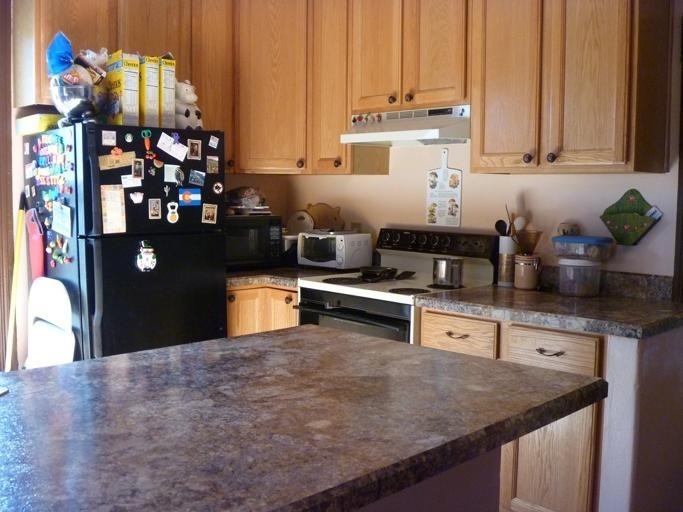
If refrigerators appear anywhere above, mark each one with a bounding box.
[21,120,226,357]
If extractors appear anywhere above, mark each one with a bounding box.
[340,104,470,146]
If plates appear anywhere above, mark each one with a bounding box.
[228,204,271,214]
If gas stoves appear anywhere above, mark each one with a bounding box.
[297,266,489,296]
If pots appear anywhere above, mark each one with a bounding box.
[359,266,397,279]
[46,84,106,117]
[431,256,464,289]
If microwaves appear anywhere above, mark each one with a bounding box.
[226,215,282,269]
[296,231,373,270]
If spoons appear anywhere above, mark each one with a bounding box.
[494,219,506,236]
[513,217,526,234]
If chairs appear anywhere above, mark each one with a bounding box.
[23,274,75,369]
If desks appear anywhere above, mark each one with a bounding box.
[0,324,608,512]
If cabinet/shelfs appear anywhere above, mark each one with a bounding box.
[188,0,237,175]
[471,1,672,176]
[11,0,190,110]
[224,285,265,339]
[263,286,300,344]
[348,0,473,112]
[419,310,602,511]
[310,0,351,175]
[236,0,310,175]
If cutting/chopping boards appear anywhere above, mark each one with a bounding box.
[286,211,313,234]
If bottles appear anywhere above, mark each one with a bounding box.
[497,235,517,287]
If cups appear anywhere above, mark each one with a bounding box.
[513,254,542,290]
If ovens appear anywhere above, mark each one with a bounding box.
[295,278,420,347]
